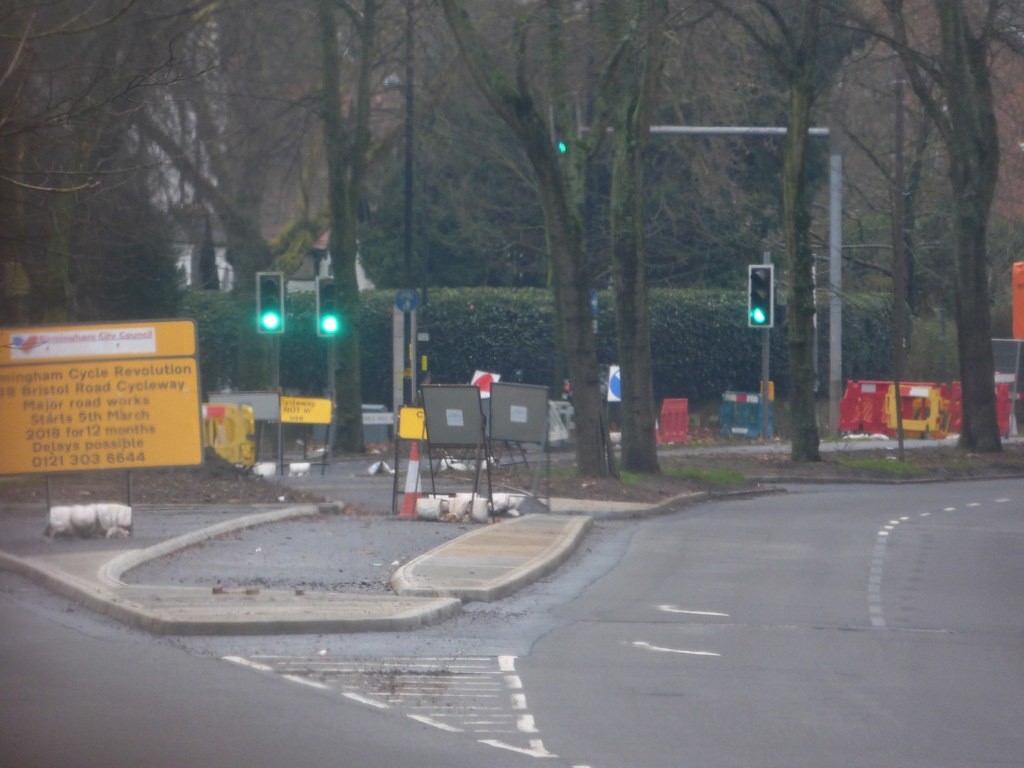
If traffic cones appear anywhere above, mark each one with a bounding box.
[395,442,424,520]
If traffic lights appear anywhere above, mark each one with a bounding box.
[550,93,582,162]
[315,274,340,337]
[254,270,286,337]
[747,264,775,329]
